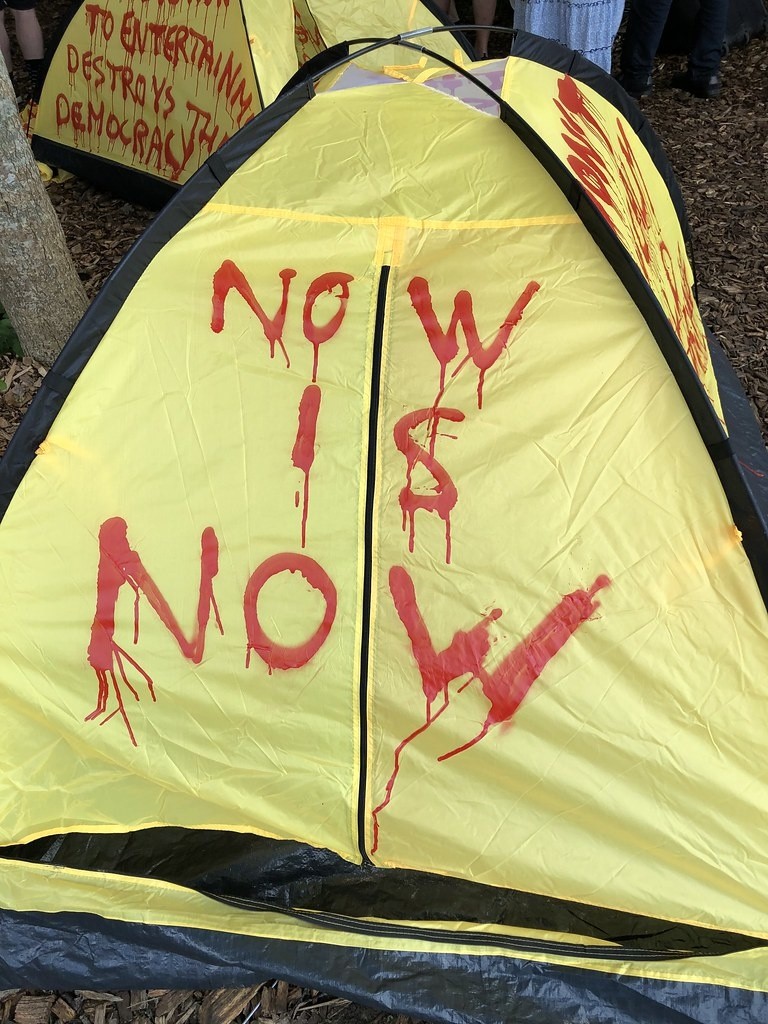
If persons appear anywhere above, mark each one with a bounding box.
[433,0,498,60]
[0,0,44,102]
[509,0,626,75]
[615,0,730,97]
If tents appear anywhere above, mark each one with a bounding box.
[30,0,473,212]
[0,20,766,1023]
